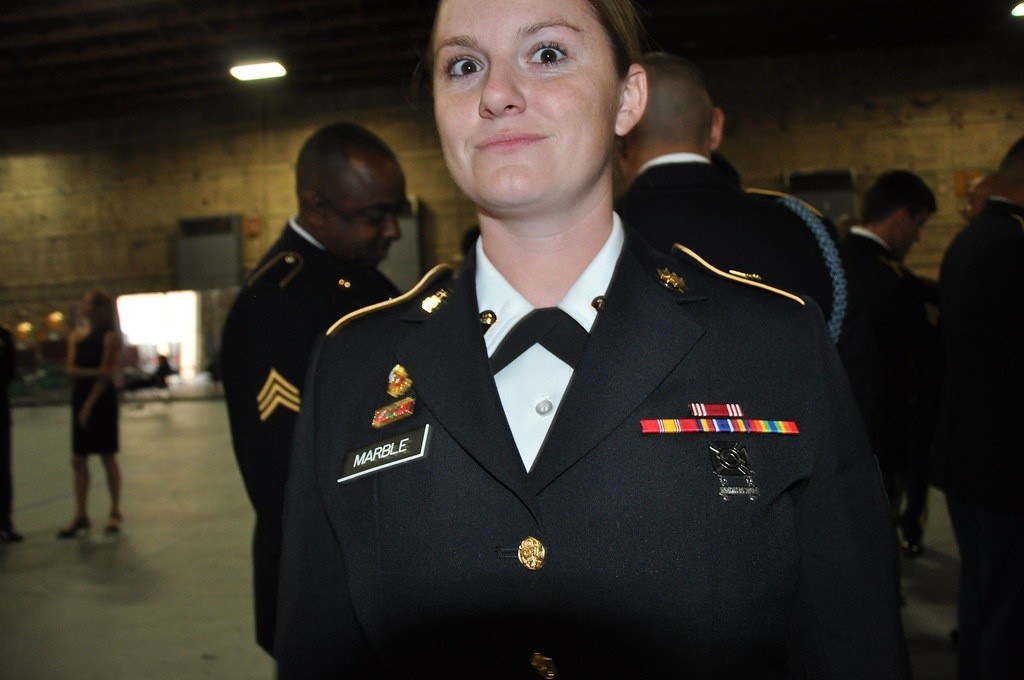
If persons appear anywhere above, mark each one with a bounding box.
[615,51,864,409]
[935,135,1024,680]
[220,123,408,659]
[56,290,122,537]
[273,0,905,680]
[0,327,24,542]
[839,168,939,484]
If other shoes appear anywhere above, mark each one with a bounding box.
[904,539,924,557]
[0,530,24,543]
[55,517,92,540]
[103,512,123,535]
